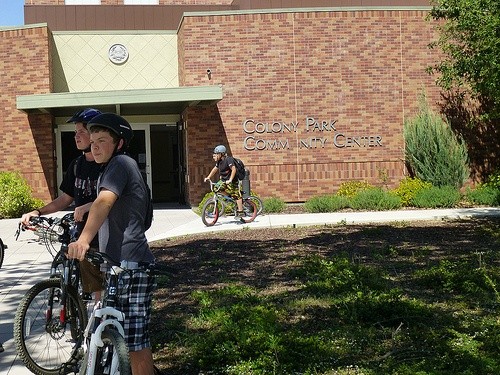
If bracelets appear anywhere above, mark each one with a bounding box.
[33,209,41,216]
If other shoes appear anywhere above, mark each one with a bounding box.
[224,195,232,204]
[234,212,246,219]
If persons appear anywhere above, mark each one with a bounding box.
[204,145,246,219]
[65,113,155,374]
[20,108,114,345]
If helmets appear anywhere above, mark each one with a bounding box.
[66,108,103,124]
[213,145,227,154]
[88,114,134,145]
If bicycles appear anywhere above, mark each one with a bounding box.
[0,237,8,271]
[13,209,118,375]
[201,178,263,227]
[61,245,178,375]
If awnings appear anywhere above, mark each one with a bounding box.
[16,84,223,116]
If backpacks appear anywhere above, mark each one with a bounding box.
[74,153,152,233]
[226,157,246,180]
